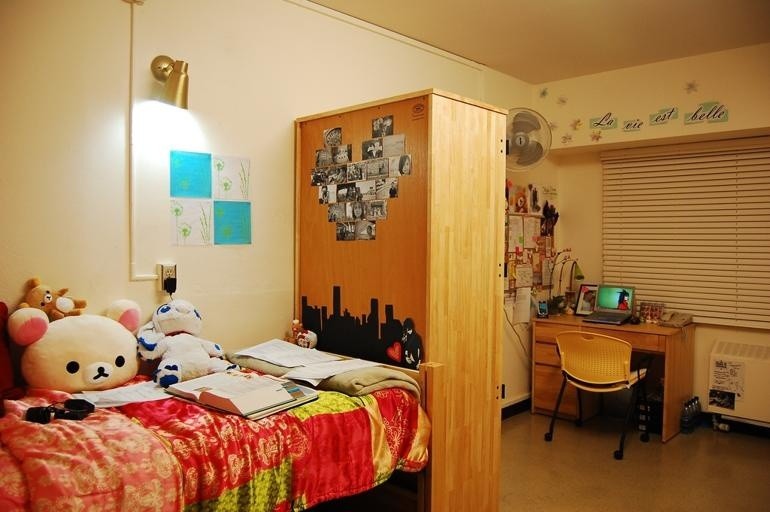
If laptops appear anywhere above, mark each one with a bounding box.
[582,283,636,326]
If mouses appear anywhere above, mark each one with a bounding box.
[630,316,640,325]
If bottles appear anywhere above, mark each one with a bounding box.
[681,395,702,433]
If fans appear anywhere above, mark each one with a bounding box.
[506,107,553,175]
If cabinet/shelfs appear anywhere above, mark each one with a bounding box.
[291,87,509,512]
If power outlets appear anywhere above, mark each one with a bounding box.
[158,264,180,292]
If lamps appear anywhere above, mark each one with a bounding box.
[149,54,192,110]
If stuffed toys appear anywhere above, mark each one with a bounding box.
[7,300,144,394]
[509,187,528,214]
[138,299,241,389]
[14,279,88,322]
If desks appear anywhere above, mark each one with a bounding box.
[530,303,697,442]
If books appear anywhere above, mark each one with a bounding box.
[164,371,321,421]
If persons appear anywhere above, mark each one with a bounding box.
[617,290,630,311]
[580,290,594,311]
[389,182,397,198]
[331,168,347,183]
[367,226,376,241]
[321,186,329,202]
[352,202,365,219]
[398,155,410,175]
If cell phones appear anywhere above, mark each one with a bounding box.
[27,406,50,424]
[64,398,94,413]
[55,408,89,419]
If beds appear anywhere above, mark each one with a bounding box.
[1,338,447,512]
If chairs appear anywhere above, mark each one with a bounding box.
[545,331,651,458]
